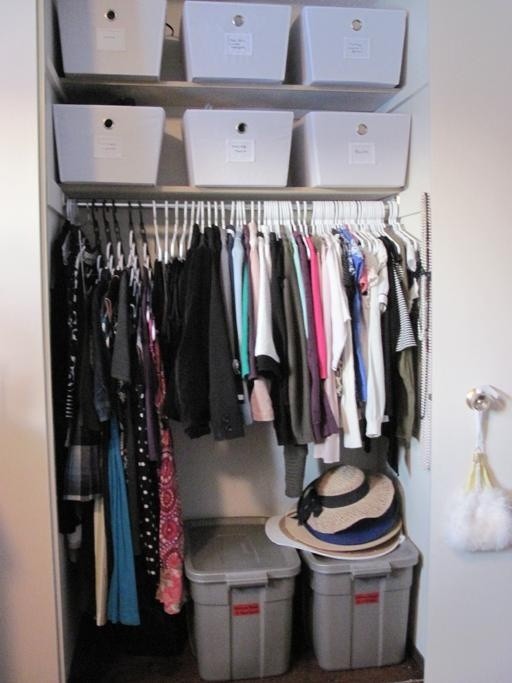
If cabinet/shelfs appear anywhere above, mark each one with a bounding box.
[48,74,406,196]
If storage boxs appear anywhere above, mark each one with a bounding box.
[293,111,412,188]
[178,0,293,83]
[293,5,408,86]
[180,107,295,188]
[300,530,420,671]
[183,515,303,682]
[53,1,168,78]
[52,100,165,185]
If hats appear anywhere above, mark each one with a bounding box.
[264,464,406,560]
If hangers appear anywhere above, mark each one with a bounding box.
[53,197,420,275]
